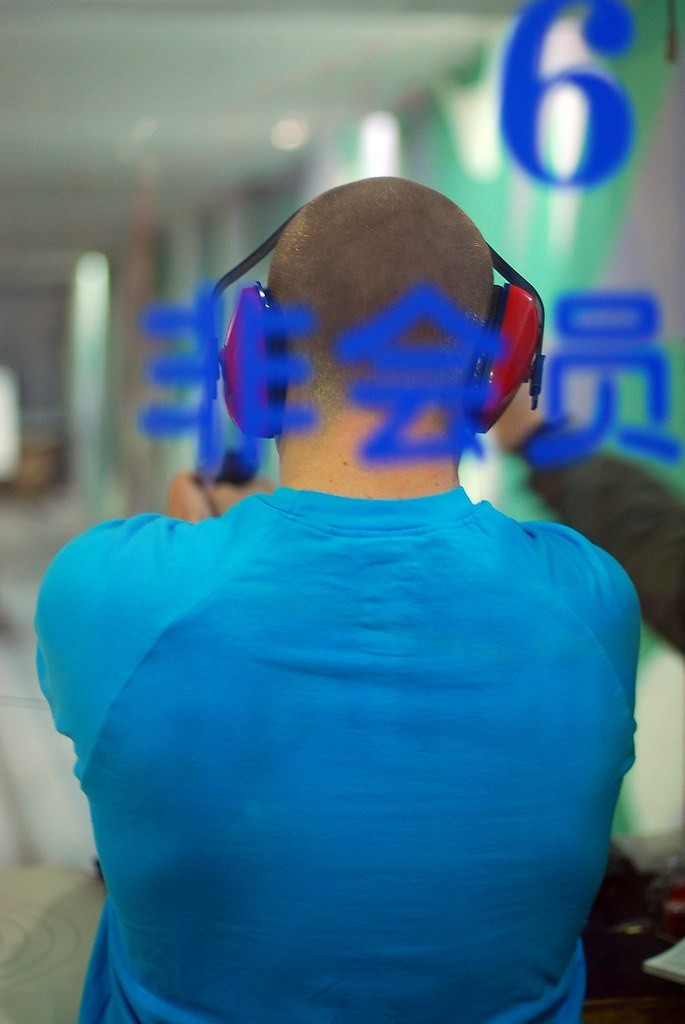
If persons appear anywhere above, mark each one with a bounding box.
[31,176,642,1024]
[493,379,685,653]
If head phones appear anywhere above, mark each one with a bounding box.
[203,205,545,438]
